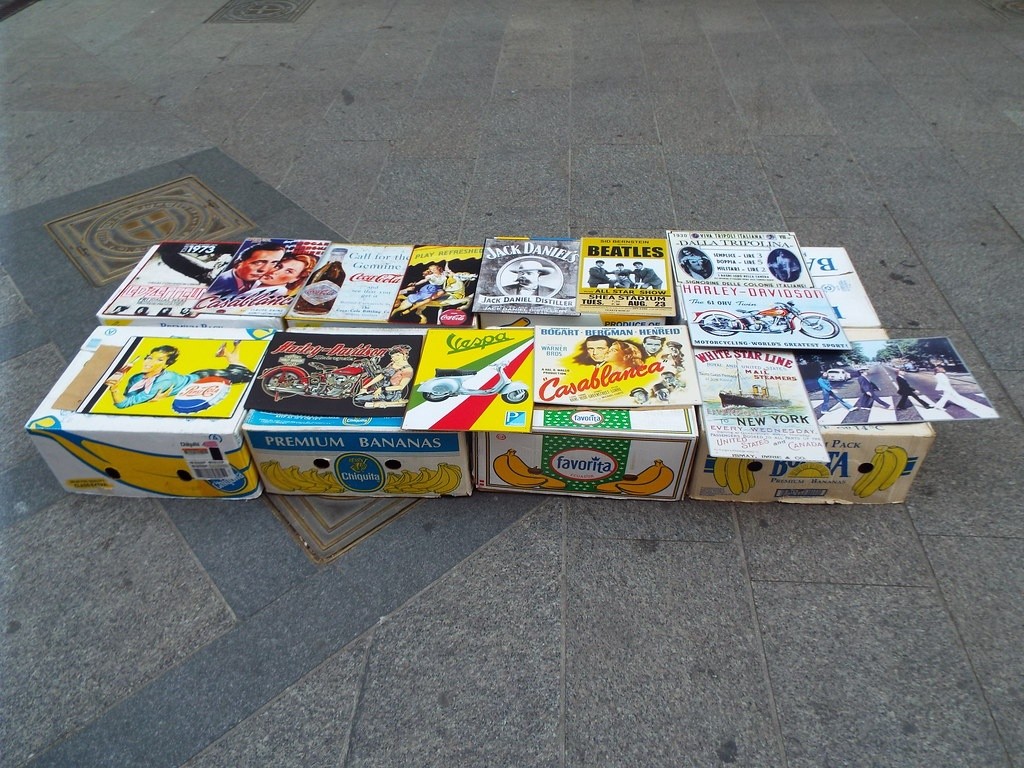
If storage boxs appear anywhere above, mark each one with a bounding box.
[27,243,936,503]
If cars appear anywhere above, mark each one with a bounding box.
[823,369,852,379]
[890,357,921,373]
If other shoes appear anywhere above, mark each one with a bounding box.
[888,406,896,410]
[862,407,871,410]
[821,411,830,414]
[849,407,858,411]
[938,408,947,410]
[897,407,904,410]
[926,406,934,408]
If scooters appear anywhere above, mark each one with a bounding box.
[415,362,538,403]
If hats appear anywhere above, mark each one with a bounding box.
[860,366,869,370]
[629,388,648,397]
[510,261,553,275]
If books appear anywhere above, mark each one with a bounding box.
[76,333,704,433]
[576,236,677,317]
[666,229,1000,462]
[103,235,575,328]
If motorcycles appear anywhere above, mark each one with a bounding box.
[257,351,409,404]
[692,301,854,343]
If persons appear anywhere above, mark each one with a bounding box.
[588,261,662,290]
[933,367,968,409]
[857,369,894,410]
[896,371,930,410]
[817,372,858,413]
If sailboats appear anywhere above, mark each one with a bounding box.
[716,356,795,409]
